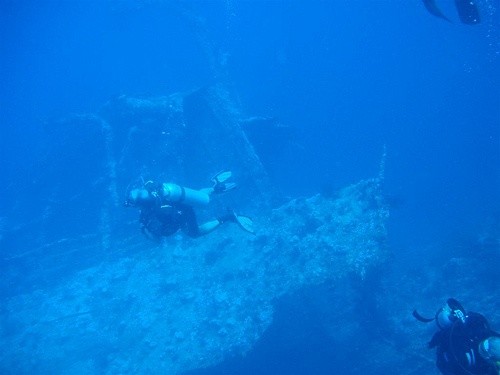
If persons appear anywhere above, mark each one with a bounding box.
[431,299,499,375]
[126,183,255,238]
